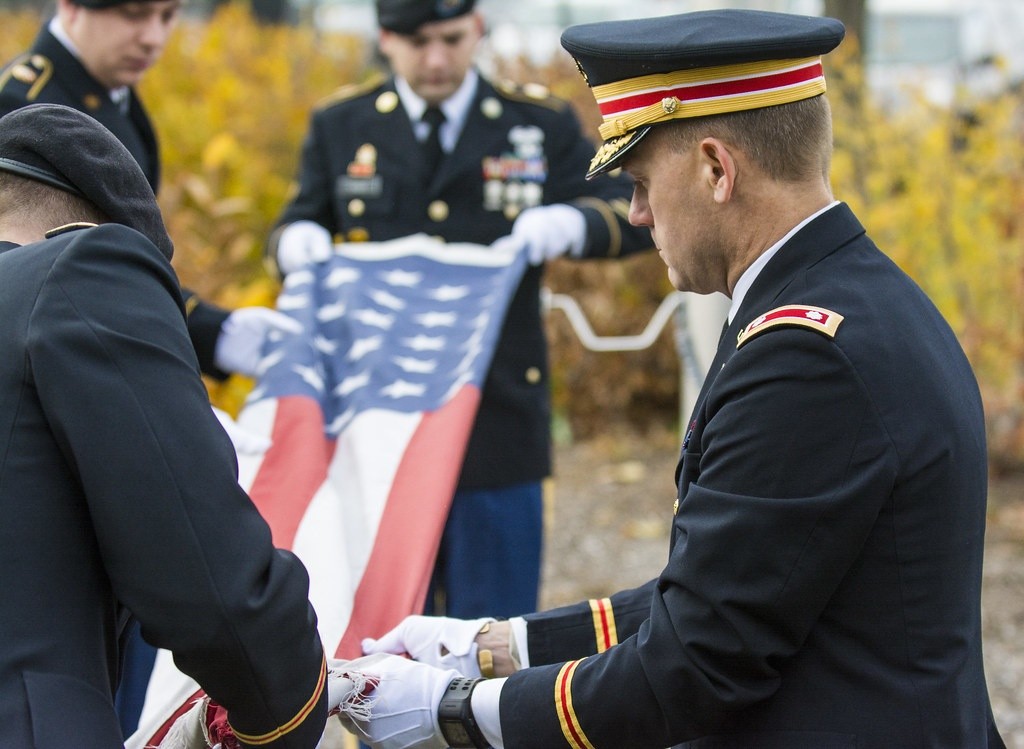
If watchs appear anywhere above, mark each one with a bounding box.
[437,677,494,749]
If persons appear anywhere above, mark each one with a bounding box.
[336,8,1001,749]
[0,102,328,749]
[272,0,659,637]
[0,0,302,474]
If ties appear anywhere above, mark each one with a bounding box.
[420,105,448,177]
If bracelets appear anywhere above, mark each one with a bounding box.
[478,623,494,681]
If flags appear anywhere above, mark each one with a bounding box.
[134,235,532,749]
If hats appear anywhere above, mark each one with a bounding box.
[560,9,846,180]
[375,0,478,34]
[0,103,174,262]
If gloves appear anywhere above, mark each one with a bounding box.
[338,656,462,749]
[275,220,331,275]
[508,203,586,265]
[360,615,498,679]
[215,308,303,377]
